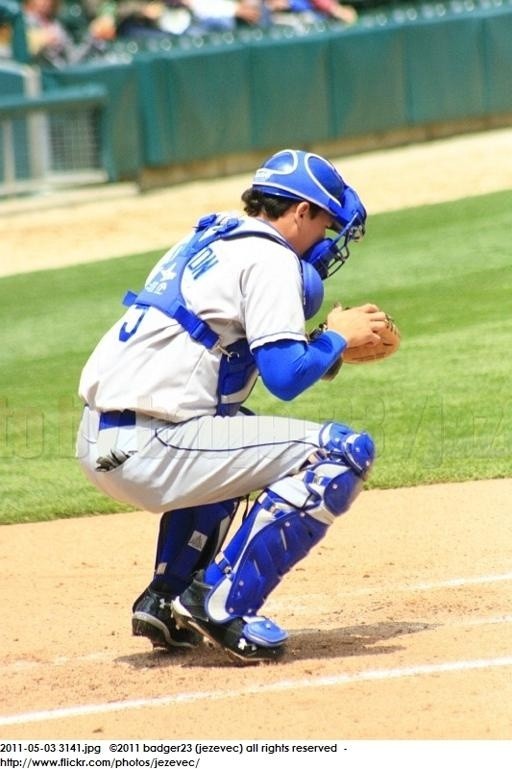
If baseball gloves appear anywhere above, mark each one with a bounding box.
[341,306,400,361]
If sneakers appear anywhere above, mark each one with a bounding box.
[131,572,205,649]
[171,580,283,664]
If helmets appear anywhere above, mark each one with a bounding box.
[252,148,367,267]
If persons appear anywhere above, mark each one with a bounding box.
[70,149,398,667]
[0,0,361,69]
